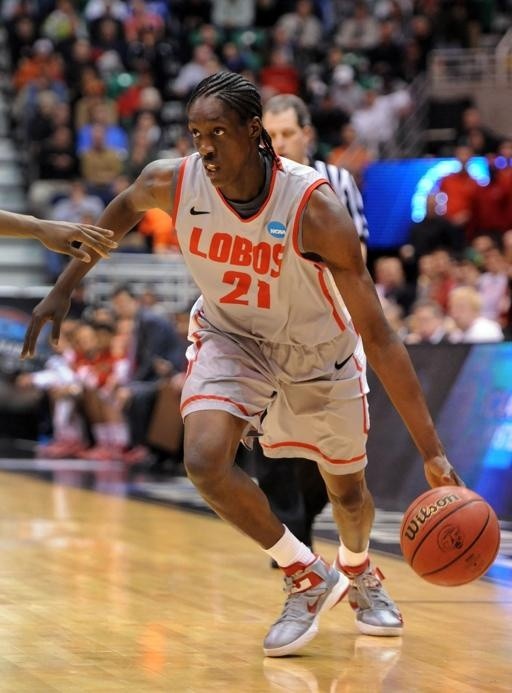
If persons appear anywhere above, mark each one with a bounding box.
[18,72,463,660]
[251,90,373,571]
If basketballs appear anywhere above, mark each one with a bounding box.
[398,486,503,587]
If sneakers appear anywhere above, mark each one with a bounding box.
[330,559,405,637]
[262,553,349,658]
